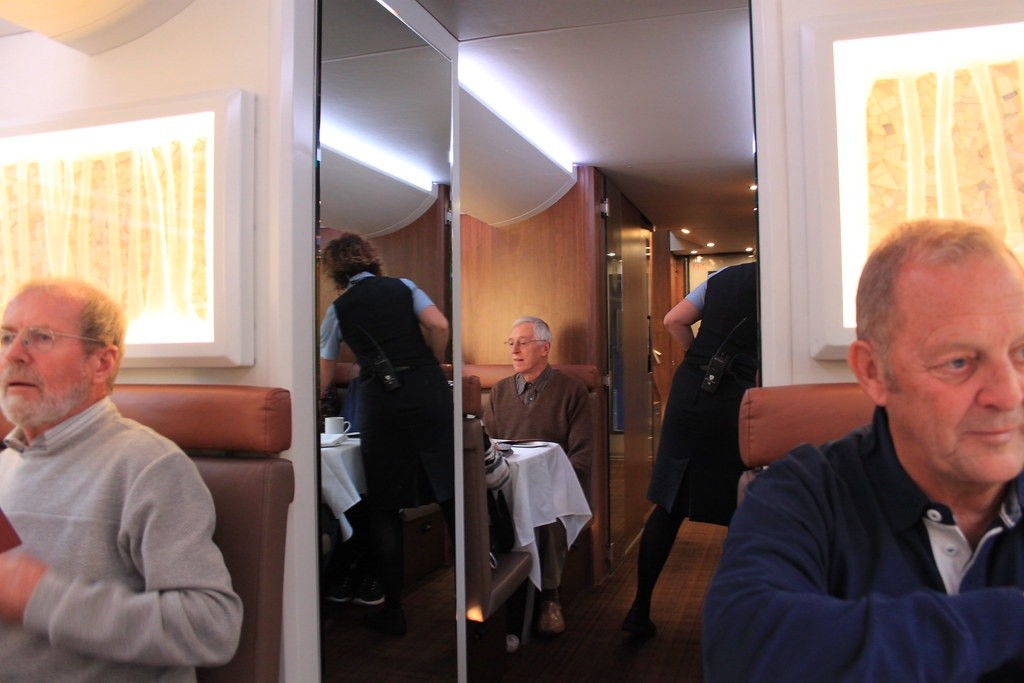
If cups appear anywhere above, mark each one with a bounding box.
[325,417,352,434]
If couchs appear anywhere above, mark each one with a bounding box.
[85,359,994,683]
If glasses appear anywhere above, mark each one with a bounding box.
[1,326,105,353]
[504,338,546,348]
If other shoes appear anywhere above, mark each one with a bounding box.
[621,612,658,632]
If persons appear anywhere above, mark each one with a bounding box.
[318,232,455,636]
[0,277,244,683]
[483,315,595,635]
[621,261,760,638]
[480,420,521,653]
[700,218,1024,683]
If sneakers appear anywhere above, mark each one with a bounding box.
[538,599,565,634]
[354,575,387,606]
[504,634,519,652]
[327,574,352,603]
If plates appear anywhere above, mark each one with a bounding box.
[513,442,547,447]
